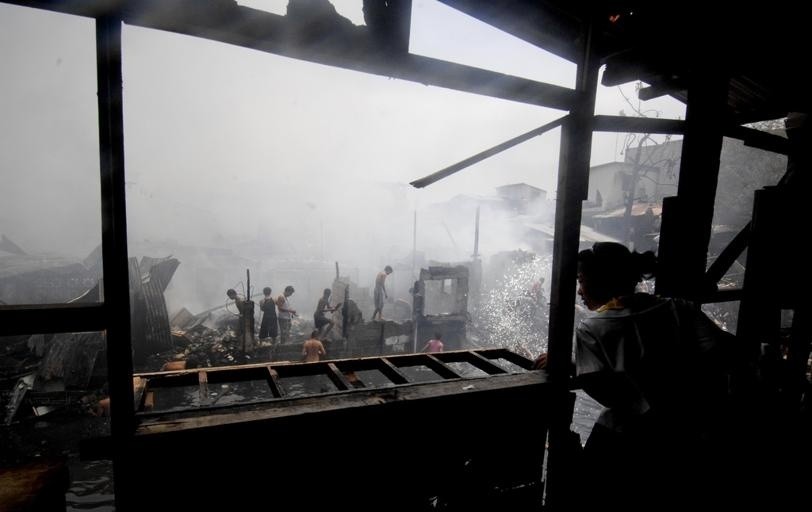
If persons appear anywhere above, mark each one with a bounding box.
[258,286,278,345]
[409,280,426,316]
[226,287,243,322]
[536,241,724,409]
[370,266,393,322]
[313,289,341,342]
[300,329,326,363]
[419,331,444,353]
[275,286,298,346]
